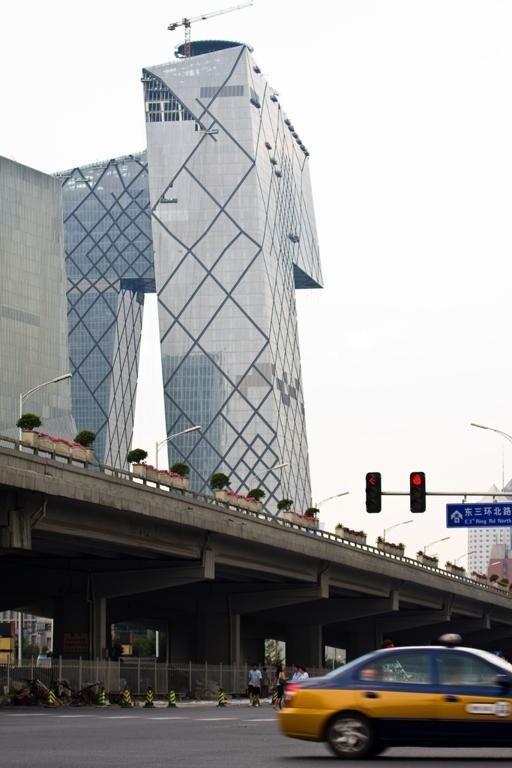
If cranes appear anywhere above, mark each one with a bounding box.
[167,1,254,59]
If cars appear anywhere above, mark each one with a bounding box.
[275,633,512,757]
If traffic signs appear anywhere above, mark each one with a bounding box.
[445,503,511,529]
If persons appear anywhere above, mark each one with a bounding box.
[248,665,270,707]
[273,664,309,710]
[381,640,409,682]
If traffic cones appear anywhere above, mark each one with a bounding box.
[98,686,177,708]
[216,687,227,708]
[44,686,56,708]
[269,688,278,705]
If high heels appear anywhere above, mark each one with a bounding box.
[273,706,282,711]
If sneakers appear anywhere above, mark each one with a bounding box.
[248,701,261,707]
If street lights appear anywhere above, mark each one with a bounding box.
[470,422,511,443]
[155,426,202,662]
[17,373,74,666]
